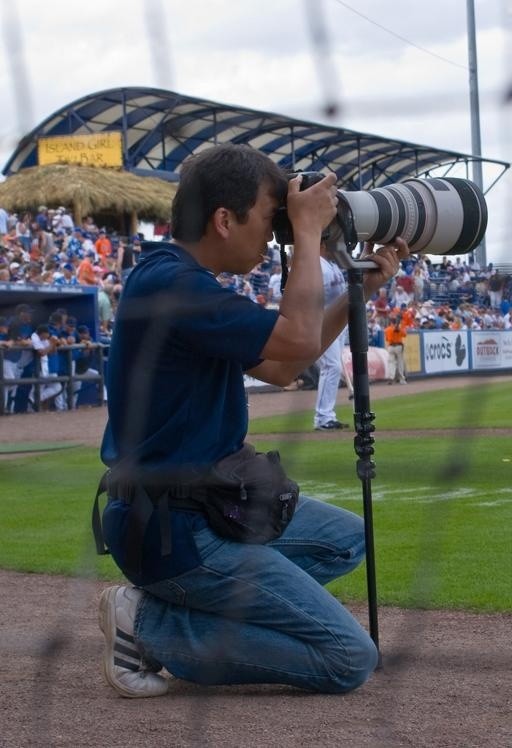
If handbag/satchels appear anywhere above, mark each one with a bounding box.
[91,441,300,545]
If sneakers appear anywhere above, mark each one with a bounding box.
[318,422,343,432]
[338,421,348,427]
[97,585,171,699]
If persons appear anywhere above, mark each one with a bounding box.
[282,235,352,429]
[366,253,511,385]
[90,142,409,699]
[0,203,144,416]
[215,244,295,309]
